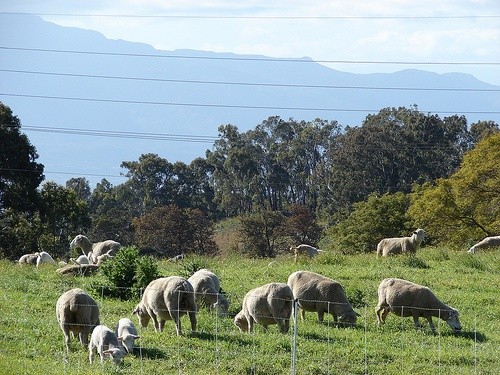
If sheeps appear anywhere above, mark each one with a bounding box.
[375,277,461,335]
[89,325,125,368]
[467,236,500,253]
[19,235,121,275]
[377,228,429,256]
[234,282,294,334]
[291,244,319,263]
[55,288,100,353]
[287,270,362,326]
[115,318,140,353]
[131,275,196,336]
[189,269,230,318]
